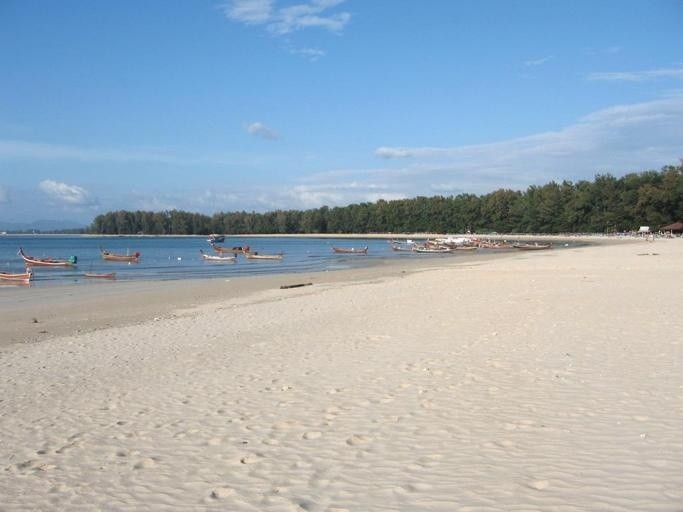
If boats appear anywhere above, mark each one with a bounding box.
[199,242,285,263]
[1,245,139,285]
[333,245,368,253]
[390,236,551,254]
